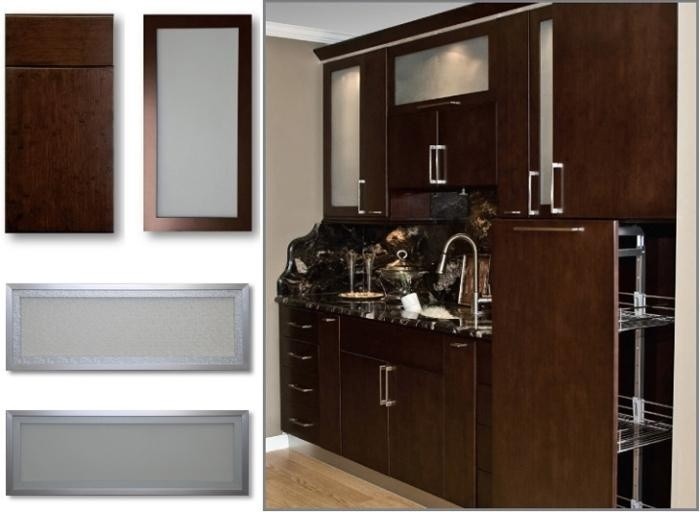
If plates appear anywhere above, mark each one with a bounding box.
[338,291,385,301]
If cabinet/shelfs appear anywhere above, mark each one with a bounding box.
[488,217,674,509]
[334,310,477,503]
[386,99,500,224]
[489,0,678,222]
[318,48,388,230]
[390,26,498,112]
[275,297,342,457]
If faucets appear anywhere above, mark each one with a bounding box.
[435,232,486,315]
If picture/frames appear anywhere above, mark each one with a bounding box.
[138,10,258,235]
[4,408,252,498]
[3,280,253,378]
